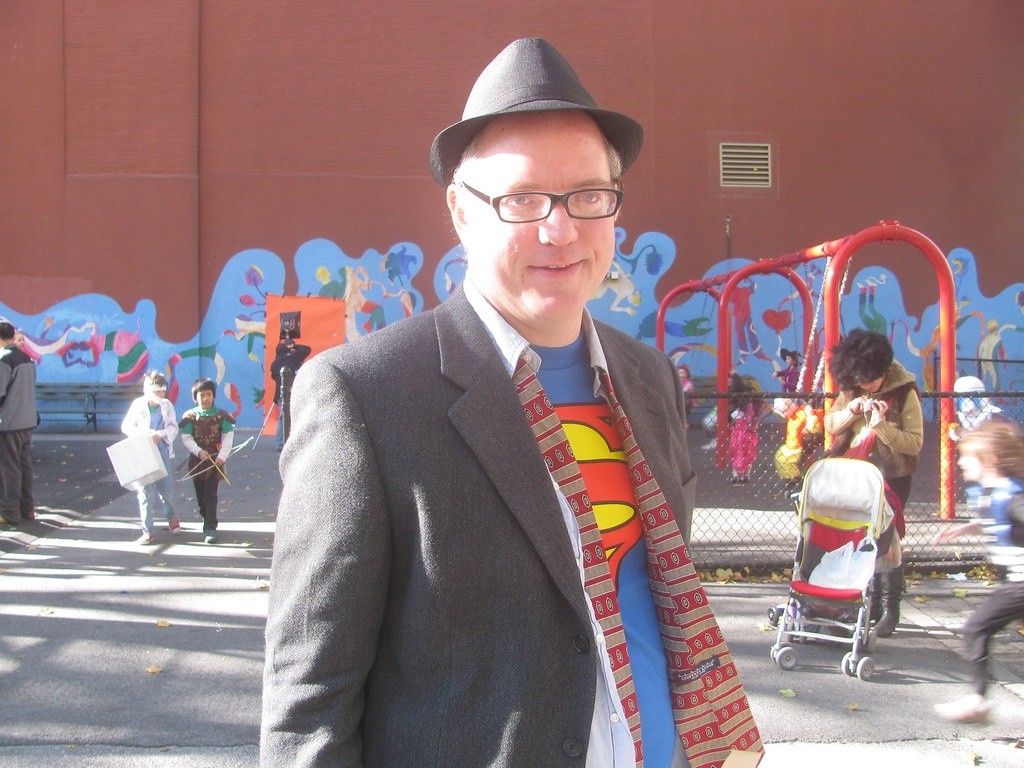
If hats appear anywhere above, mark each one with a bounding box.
[428,36,642,187]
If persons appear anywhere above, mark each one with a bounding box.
[675,330,1024,723]
[179,377,237,542]
[269,340,312,450]
[0,317,38,523]
[120,372,181,544]
[257,36,763,766]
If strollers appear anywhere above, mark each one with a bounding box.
[770,450,896,680]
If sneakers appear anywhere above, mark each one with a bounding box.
[205,528,217,543]
[137,532,154,545]
[168,516,180,534]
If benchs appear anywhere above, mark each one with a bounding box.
[678,376,759,427]
[32,382,144,433]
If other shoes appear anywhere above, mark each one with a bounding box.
[934,698,991,723]
[0,516,12,524]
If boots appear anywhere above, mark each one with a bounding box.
[870,572,882,624]
[871,563,903,637]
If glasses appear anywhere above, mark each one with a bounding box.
[459,178,624,223]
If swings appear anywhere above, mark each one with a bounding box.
[773,254,853,480]
[685,283,720,419]
